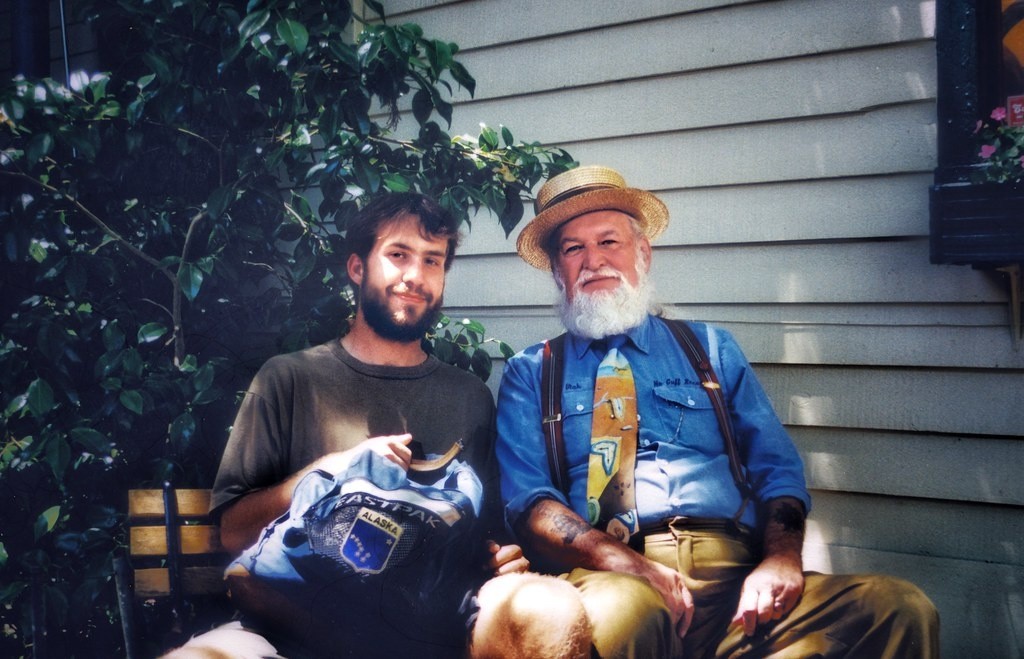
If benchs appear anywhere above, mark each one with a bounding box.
[113,486,223,659]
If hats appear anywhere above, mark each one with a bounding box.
[516,167,670,271]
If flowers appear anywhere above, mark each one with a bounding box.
[971,108,1024,185]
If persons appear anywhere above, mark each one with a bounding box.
[149,192,512,659]
[497,164,943,659]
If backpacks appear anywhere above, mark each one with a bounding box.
[222,445,493,659]
